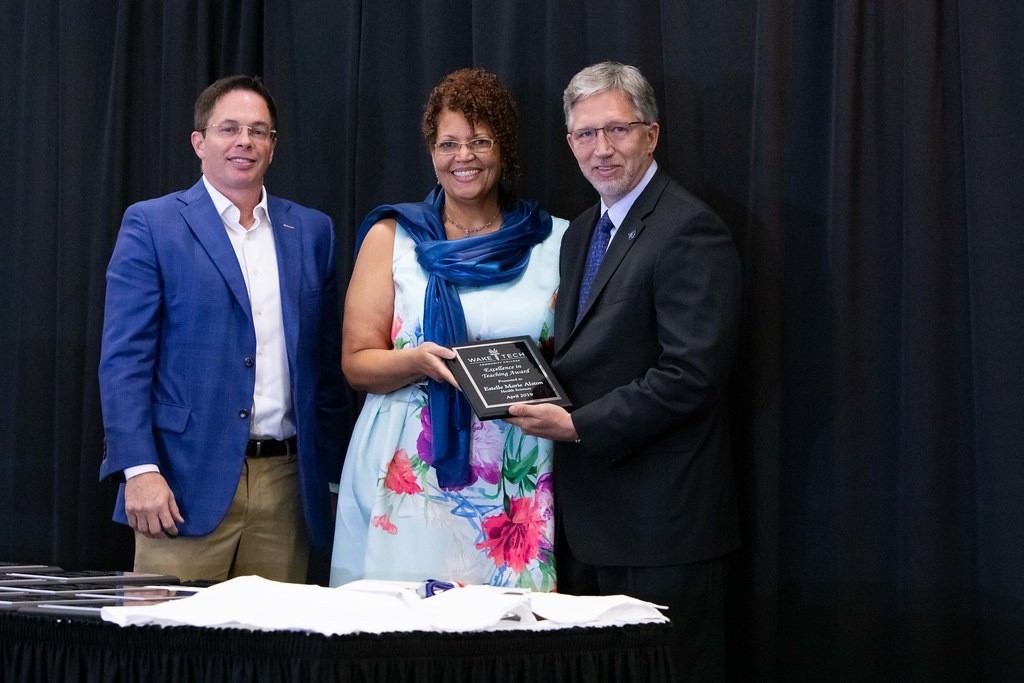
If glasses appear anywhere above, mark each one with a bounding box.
[199,121,276,140]
[569,120,652,143]
[431,138,499,153]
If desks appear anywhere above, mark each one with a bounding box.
[0,561,679,683]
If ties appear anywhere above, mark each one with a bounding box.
[576,210,615,323]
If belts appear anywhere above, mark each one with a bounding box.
[246,436,298,459]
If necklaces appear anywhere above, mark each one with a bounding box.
[444,204,500,238]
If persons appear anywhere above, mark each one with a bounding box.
[502,61,742,683]
[328,67,571,594]
[98,74,339,583]
